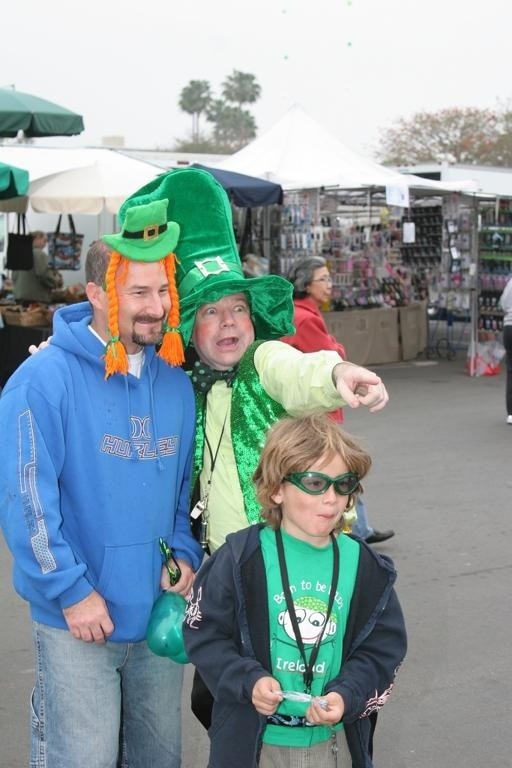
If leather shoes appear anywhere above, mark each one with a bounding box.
[365,530,395,543]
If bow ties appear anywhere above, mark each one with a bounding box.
[189,361,236,394]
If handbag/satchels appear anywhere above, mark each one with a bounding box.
[7,213,34,270]
[47,214,83,269]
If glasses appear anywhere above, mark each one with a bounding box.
[283,472,360,495]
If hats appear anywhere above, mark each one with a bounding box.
[121,168,296,346]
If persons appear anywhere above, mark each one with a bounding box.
[180,411,408,766]
[184,287,390,731]
[498,278,512,425]
[0,239,206,766]
[11,231,63,308]
[277,254,395,544]
